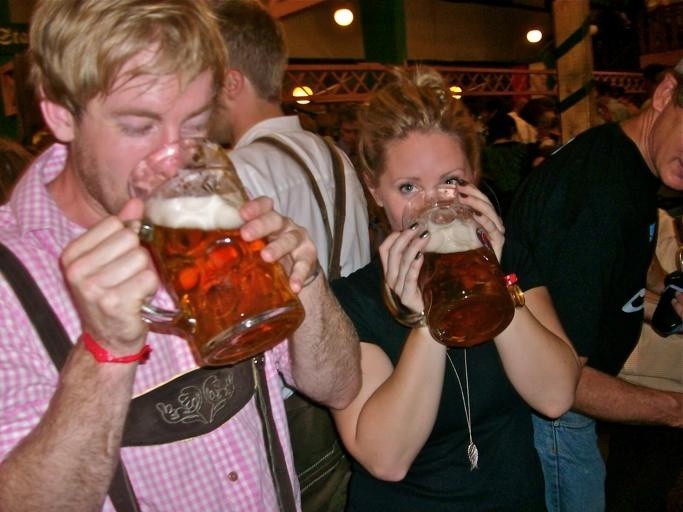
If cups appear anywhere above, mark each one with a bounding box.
[122,138,306,370]
[380,183,517,351]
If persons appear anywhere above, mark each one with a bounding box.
[0,0,683,512]
